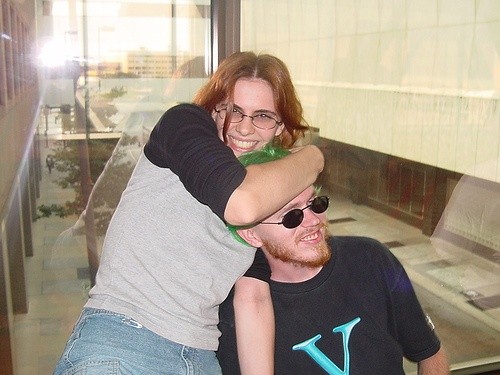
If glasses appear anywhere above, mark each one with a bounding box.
[212,104,284,131]
[255,196,330,229]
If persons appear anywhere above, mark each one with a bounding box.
[54,51,324,375]
[50,56,205,269]
[214,143,449,375]
